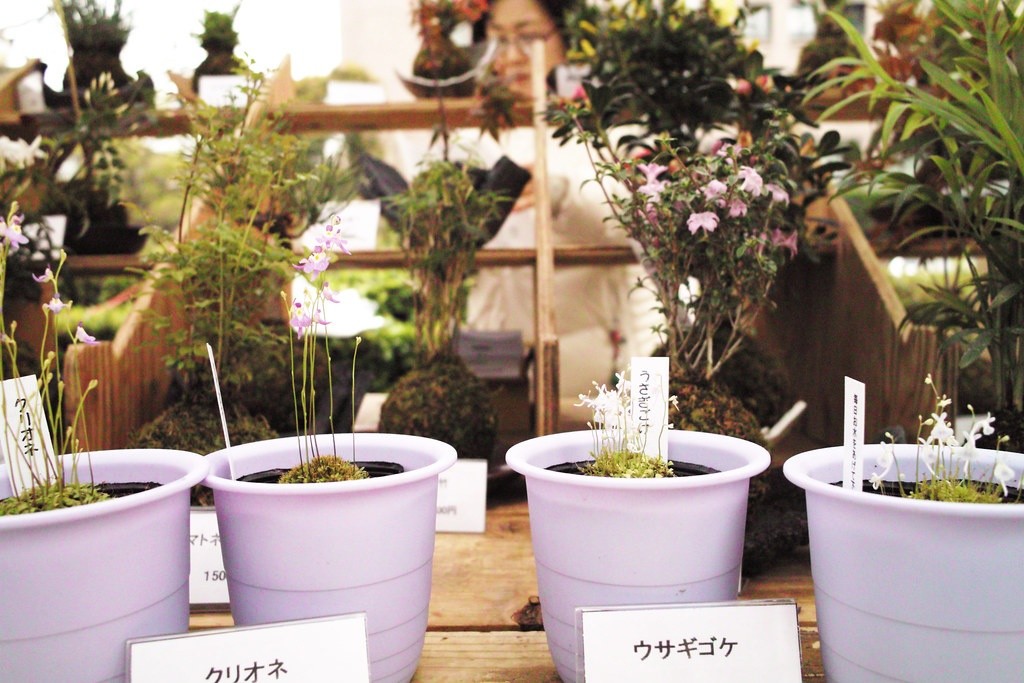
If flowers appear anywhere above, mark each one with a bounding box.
[276,220,368,482]
[548,72,854,390]
[0,200,111,516]
[868,372,1024,503]
[571,370,680,477]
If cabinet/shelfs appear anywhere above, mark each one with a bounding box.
[22,99,971,275]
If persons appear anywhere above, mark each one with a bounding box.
[456,0,689,402]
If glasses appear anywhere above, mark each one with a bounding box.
[488,24,563,57]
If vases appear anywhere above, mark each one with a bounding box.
[200,433,457,683]
[0,447,209,683]
[782,443,1024,683]
[505,430,771,683]
